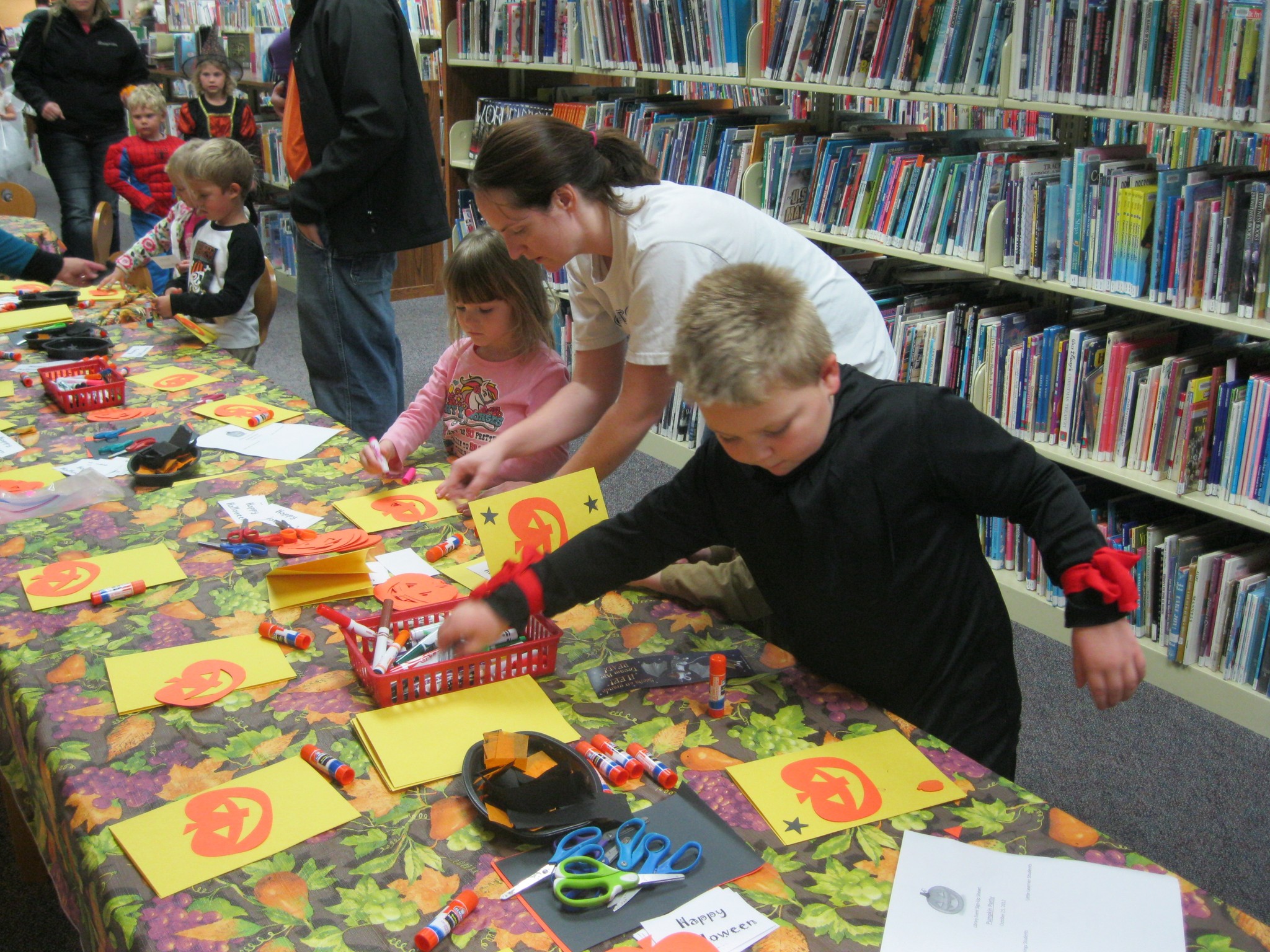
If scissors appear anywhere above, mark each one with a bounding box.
[551,815,649,867]
[551,855,686,908]
[500,826,606,900]
[108,437,157,458]
[197,541,268,558]
[551,837,632,915]
[99,436,146,453]
[93,424,141,439]
[614,818,667,894]
[226,518,259,543]
[605,831,703,914]
[178,394,226,411]
[274,519,317,539]
[221,533,296,545]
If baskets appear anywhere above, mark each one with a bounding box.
[338,594,564,708]
[37,358,126,414]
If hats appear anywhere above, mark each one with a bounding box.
[181,21,244,82]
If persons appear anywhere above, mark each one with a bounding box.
[625,544,790,653]
[272,0,452,443]
[0,86,33,177]
[97,138,259,296]
[436,264,1146,784]
[22,0,55,36]
[178,59,263,228]
[150,138,265,366]
[359,221,570,501]
[13,0,150,285]
[0,229,108,287]
[437,114,898,516]
[104,82,188,297]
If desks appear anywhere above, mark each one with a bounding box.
[0,217,1270,952]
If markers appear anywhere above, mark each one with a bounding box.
[316,597,545,703]
[54,370,122,406]
[369,436,391,478]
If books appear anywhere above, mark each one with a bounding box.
[116,19,148,49]
[165,0,299,277]
[397,0,445,161]
[825,242,1270,518]
[446,0,1270,320]
[3,27,22,49]
[975,467,1270,692]
[548,306,704,452]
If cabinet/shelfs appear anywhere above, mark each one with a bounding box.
[147,0,1270,738]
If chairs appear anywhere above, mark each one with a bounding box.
[0,182,278,367]
